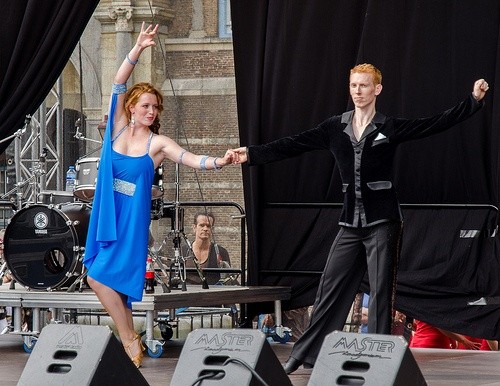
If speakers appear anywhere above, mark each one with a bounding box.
[169,329,294,386]
[17,324,150,386]
[306,330,427,386]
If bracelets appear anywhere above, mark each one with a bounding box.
[179,149,187,165]
[200,156,210,170]
[213,157,222,170]
[126,54,138,65]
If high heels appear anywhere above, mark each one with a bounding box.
[124,333,145,368]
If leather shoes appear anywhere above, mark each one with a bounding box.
[283,357,314,374]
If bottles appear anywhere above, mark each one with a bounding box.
[146,259,154,294]
[66,166,77,192]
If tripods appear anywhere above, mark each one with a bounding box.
[151,162,209,292]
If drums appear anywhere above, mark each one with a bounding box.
[150,197,164,221]
[151,163,164,197]
[3,202,93,290]
[72,157,101,201]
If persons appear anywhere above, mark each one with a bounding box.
[232,62,490,375]
[81,21,239,368]
[408,317,491,350]
[161,212,231,285]
[353,293,370,334]
